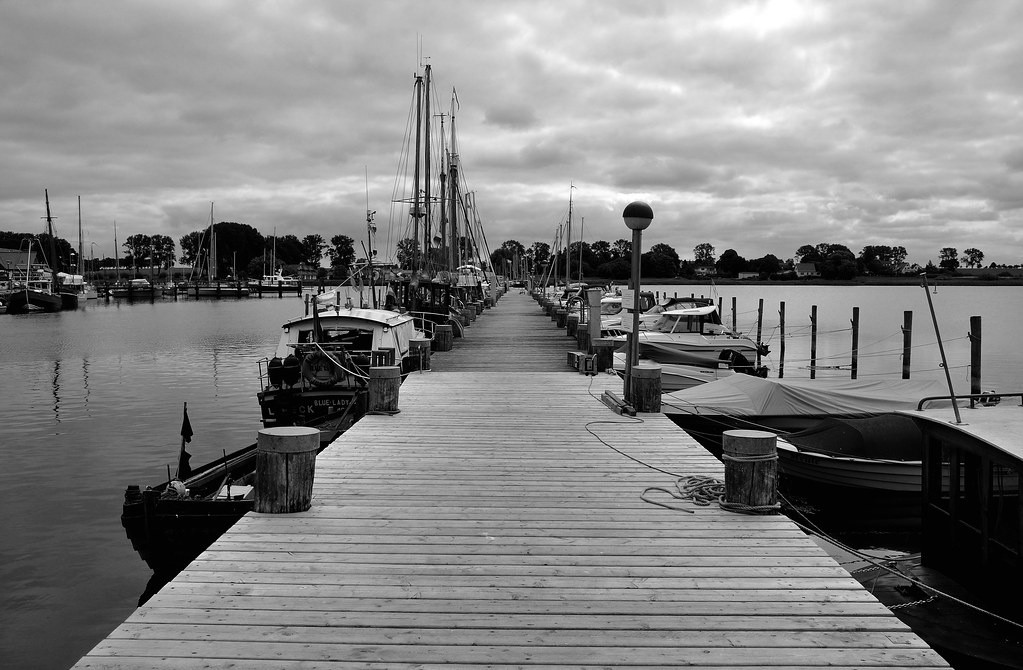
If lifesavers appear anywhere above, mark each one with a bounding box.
[302,350,343,387]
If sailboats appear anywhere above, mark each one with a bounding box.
[526,182,653,328]
[359,32,503,349]
[0,188,300,315]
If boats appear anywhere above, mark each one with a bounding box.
[594,294,1023,670]
[120,307,434,574]
